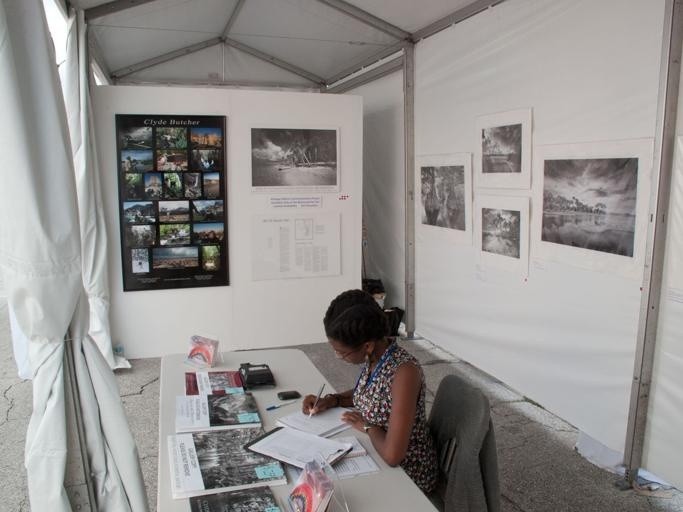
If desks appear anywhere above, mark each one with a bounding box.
[158,346,437,512]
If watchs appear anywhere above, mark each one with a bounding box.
[325,392,339,407]
[363,422,372,433]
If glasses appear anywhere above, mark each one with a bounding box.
[334,348,358,361]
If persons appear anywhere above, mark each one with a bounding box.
[303,290,439,500]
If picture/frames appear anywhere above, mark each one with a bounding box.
[114,112,229,294]
[412,149,474,239]
[247,124,341,195]
[470,189,532,280]
[534,140,654,268]
[472,106,533,190]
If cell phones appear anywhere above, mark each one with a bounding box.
[277,390,302,401]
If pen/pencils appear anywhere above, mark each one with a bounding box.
[266,400,296,411]
[309,383,325,418]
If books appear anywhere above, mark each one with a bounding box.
[277,409,353,437]
[185,371,244,395]
[284,437,377,482]
[190,486,280,511]
[175,394,262,433]
[166,427,289,498]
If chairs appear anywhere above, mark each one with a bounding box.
[427,372,489,512]
